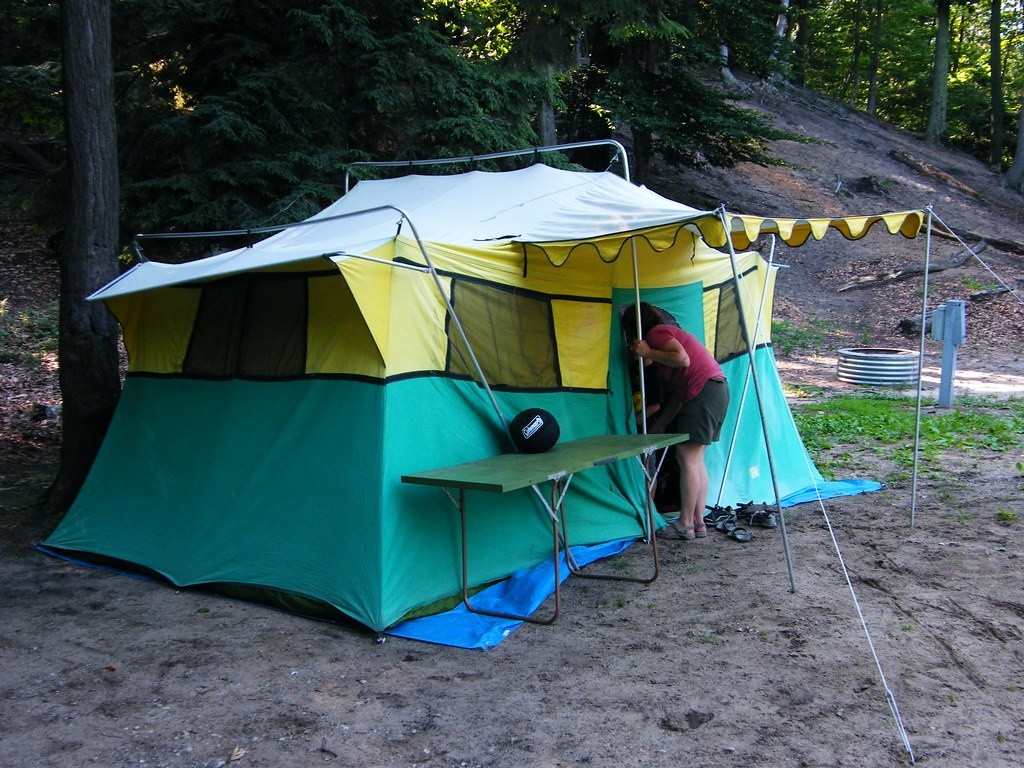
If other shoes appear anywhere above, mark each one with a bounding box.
[734,500,778,528]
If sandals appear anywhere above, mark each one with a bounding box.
[655,521,707,539]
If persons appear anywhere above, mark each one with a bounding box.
[621,300,732,542]
[626,358,665,499]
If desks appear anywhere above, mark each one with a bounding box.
[401,435,690,626]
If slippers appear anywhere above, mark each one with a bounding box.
[716,520,750,541]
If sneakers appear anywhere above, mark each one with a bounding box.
[703,504,737,526]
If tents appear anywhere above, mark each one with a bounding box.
[34,160,923,637]
